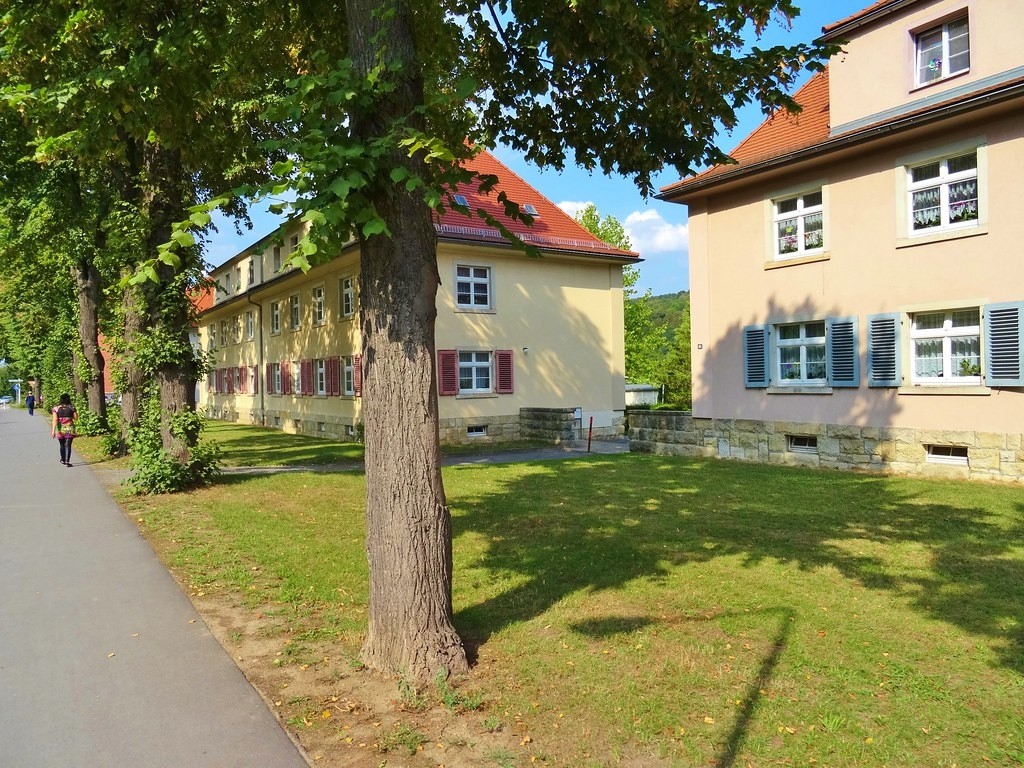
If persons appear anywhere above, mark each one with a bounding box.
[51,393,78,467]
[25,392,36,416]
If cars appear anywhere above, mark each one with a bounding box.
[0,396,14,406]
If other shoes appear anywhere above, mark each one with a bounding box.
[66,464,73,467]
[60,459,64,465]
